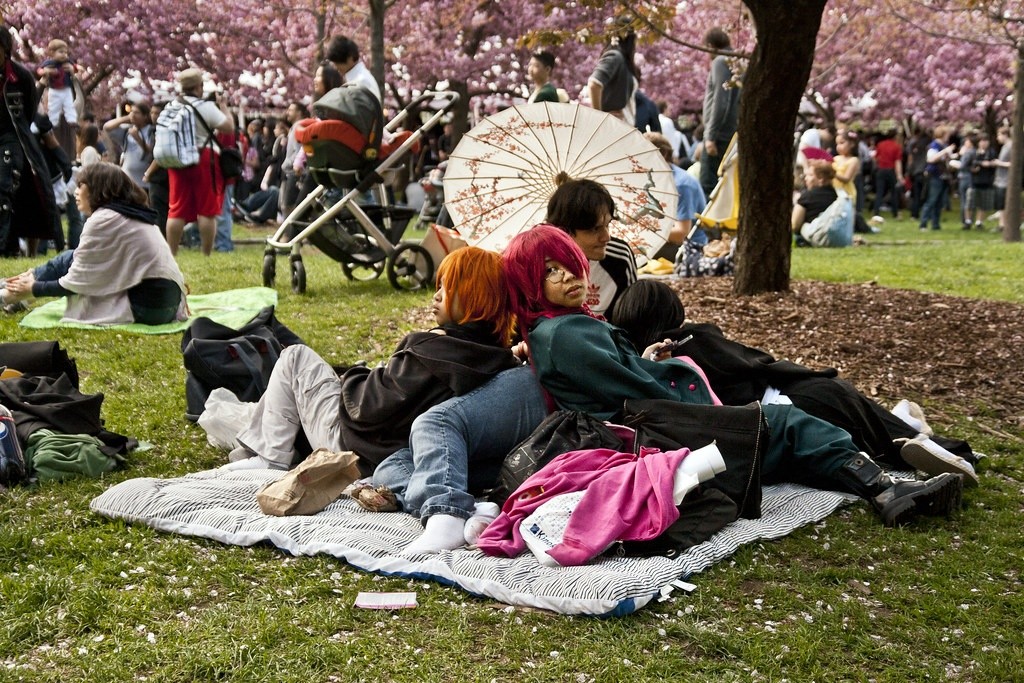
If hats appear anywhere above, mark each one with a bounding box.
[48,39,67,50]
[178,68,202,88]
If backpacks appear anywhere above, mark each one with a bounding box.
[153,98,215,168]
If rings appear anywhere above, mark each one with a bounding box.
[13,292,16,296]
[14,288,16,291]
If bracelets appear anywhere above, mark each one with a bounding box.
[143,172,151,180]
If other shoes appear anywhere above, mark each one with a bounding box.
[0,276,26,314]
[900,434,978,487]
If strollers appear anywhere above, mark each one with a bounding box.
[260,85,461,294]
[673,122,807,271]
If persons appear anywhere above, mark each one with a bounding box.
[0,162,188,326]
[235,180,983,557]
[0,26,1024,256]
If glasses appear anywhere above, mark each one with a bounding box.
[545,270,565,284]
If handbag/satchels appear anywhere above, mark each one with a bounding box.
[404,222,468,284]
[1,341,79,394]
[180,307,305,420]
[488,411,625,509]
[625,399,770,520]
[800,187,854,246]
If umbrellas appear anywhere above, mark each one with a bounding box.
[443,100,679,270]
[441,101,679,270]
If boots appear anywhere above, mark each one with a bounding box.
[834,451,964,527]
